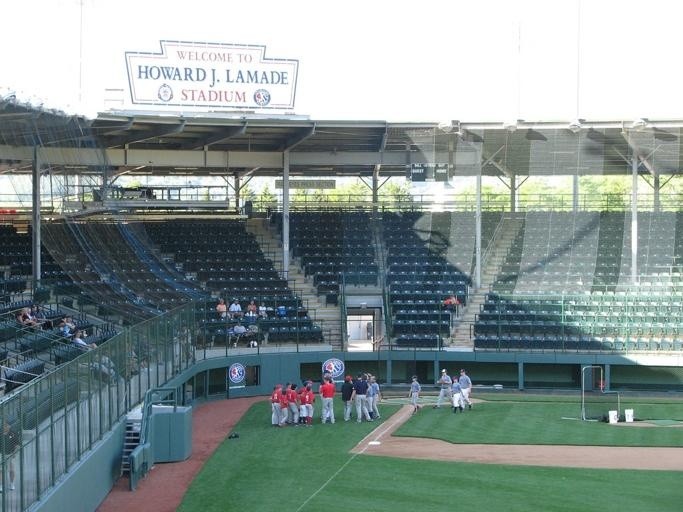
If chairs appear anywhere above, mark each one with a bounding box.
[266,211,505,346]
[0,210,324,436]
[470,213,682,350]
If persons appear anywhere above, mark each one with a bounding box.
[407,376,422,415]
[433,367,473,413]
[269,373,381,425]
[14,304,118,378]
[215,298,271,347]
